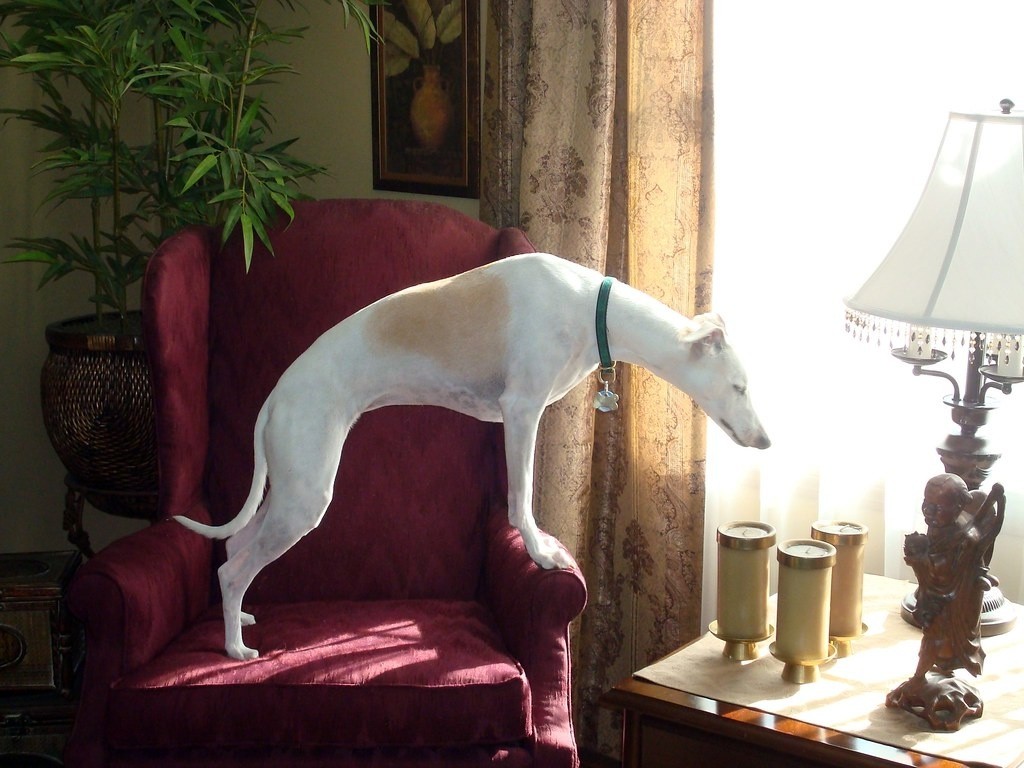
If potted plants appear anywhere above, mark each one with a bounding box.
[0,0,334,562]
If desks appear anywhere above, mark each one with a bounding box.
[597,571,1024,768]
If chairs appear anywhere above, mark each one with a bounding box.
[65,198,592,768]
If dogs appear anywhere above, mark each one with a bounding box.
[171,252,773,659]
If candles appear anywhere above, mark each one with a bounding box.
[812,521,869,635]
[776,539,837,658]
[717,520,776,638]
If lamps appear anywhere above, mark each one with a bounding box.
[832,98,1024,640]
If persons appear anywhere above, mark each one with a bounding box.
[902,473,1006,629]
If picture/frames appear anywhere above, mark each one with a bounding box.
[369,0,481,201]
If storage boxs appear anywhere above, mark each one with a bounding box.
[0,550,83,692]
